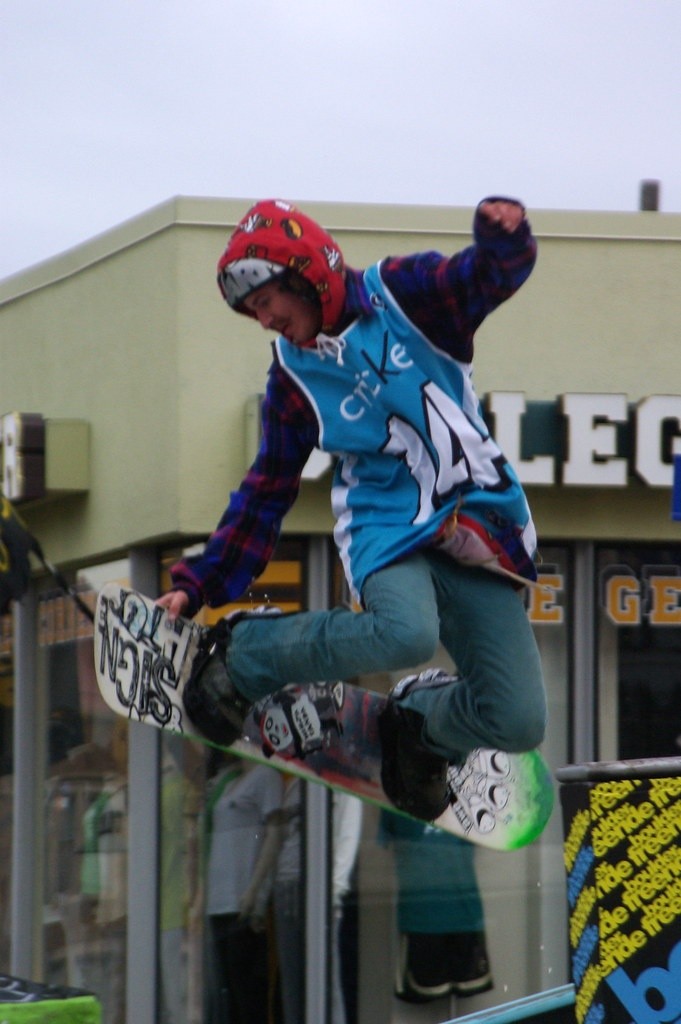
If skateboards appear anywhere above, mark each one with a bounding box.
[91,581,557,856]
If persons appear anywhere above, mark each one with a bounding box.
[150,198,548,821]
[188,752,287,1024]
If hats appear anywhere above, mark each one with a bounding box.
[220,258,284,308]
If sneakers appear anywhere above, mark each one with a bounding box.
[184,610,253,746]
[380,669,452,820]
